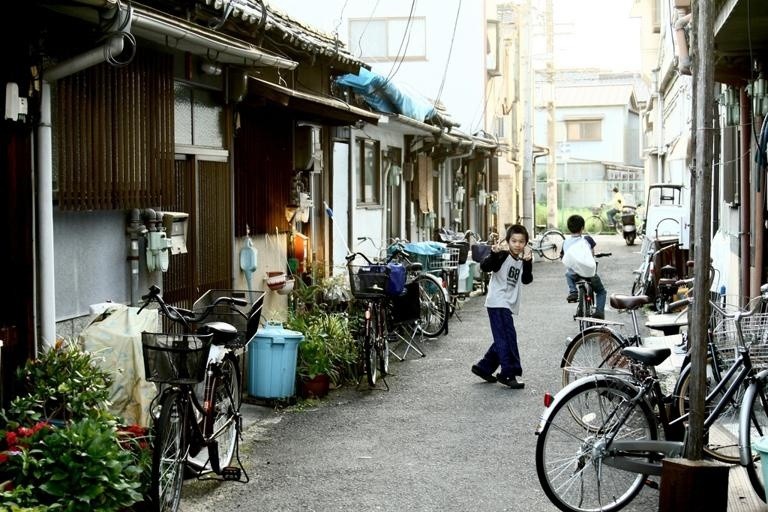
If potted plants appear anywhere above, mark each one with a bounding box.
[286,310,354,399]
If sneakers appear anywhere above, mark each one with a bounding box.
[495,373,525,389]
[472,366,496,383]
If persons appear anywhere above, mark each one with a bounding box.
[560,214,608,319]
[606,187,623,227]
[470,224,533,389]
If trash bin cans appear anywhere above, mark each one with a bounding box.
[247,320,305,411]
[465,262,477,293]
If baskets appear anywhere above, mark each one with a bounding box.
[446,243,467,263]
[192,289,266,347]
[418,248,459,269]
[143,332,214,386]
[348,264,392,299]
[712,312,768,368]
[472,245,490,262]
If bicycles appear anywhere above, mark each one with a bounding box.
[136,284,266,511]
[498,214,565,262]
[536,293,768,512]
[559,260,768,464]
[586,203,624,236]
[345,226,475,396]
[568,252,612,344]
[638,219,647,240]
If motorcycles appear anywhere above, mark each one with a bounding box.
[631,237,684,313]
[617,199,642,246]
[468,229,499,294]
